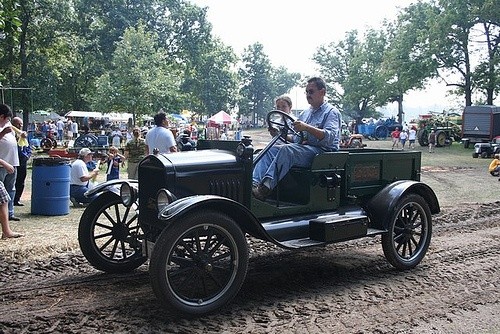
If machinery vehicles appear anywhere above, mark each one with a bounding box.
[418,121,453,147]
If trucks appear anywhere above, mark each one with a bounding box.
[462,105,500,148]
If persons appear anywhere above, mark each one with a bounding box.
[0,103,20,222]
[232,116,250,130]
[100,146,125,181]
[271,95,296,133]
[121,127,146,180]
[219,129,227,140]
[106,124,121,146]
[31,119,78,140]
[145,113,176,154]
[428,130,436,153]
[252,77,342,202]
[178,118,196,126]
[70,148,99,207]
[0,159,23,240]
[12,117,32,206]
[349,124,354,134]
[176,128,195,151]
[391,124,417,149]
[489,154,500,175]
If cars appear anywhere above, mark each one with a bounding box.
[77,110,441,316]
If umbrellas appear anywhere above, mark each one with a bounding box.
[209,110,232,125]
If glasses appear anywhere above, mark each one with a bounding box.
[306,91,320,94]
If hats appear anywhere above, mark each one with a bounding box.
[78,147,95,156]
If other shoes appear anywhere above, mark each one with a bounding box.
[9,216,20,221]
[71,197,80,207]
[14,202,23,206]
[3,233,23,238]
[251,181,268,198]
